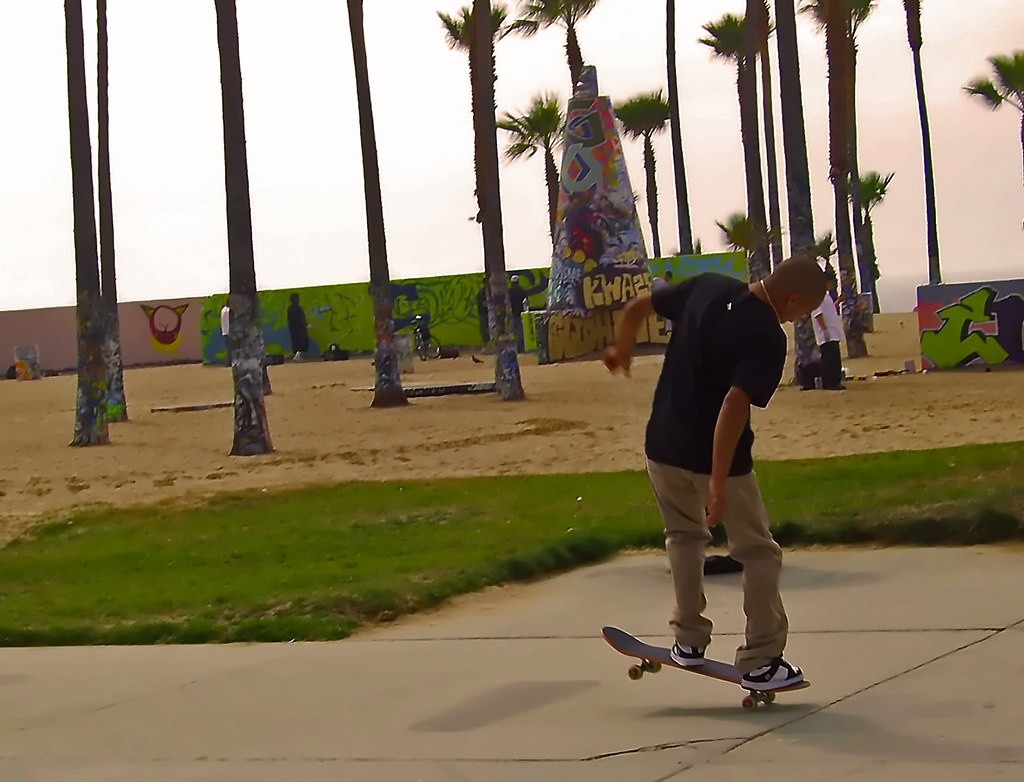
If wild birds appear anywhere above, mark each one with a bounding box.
[469,354,485,364]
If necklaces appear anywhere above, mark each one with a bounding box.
[760,280,781,323]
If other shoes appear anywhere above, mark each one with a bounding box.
[823,383,847,390]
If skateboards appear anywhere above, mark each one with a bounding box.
[600,624,812,709]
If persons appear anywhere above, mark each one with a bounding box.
[508,275,529,353]
[477,277,493,355]
[811,273,846,390]
[221,298,232,367]
[601,254,829,693]
[287,292,310,362]
[653,271,673,291]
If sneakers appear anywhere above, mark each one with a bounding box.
[742,658,806,690]
[670,640,706,666]
